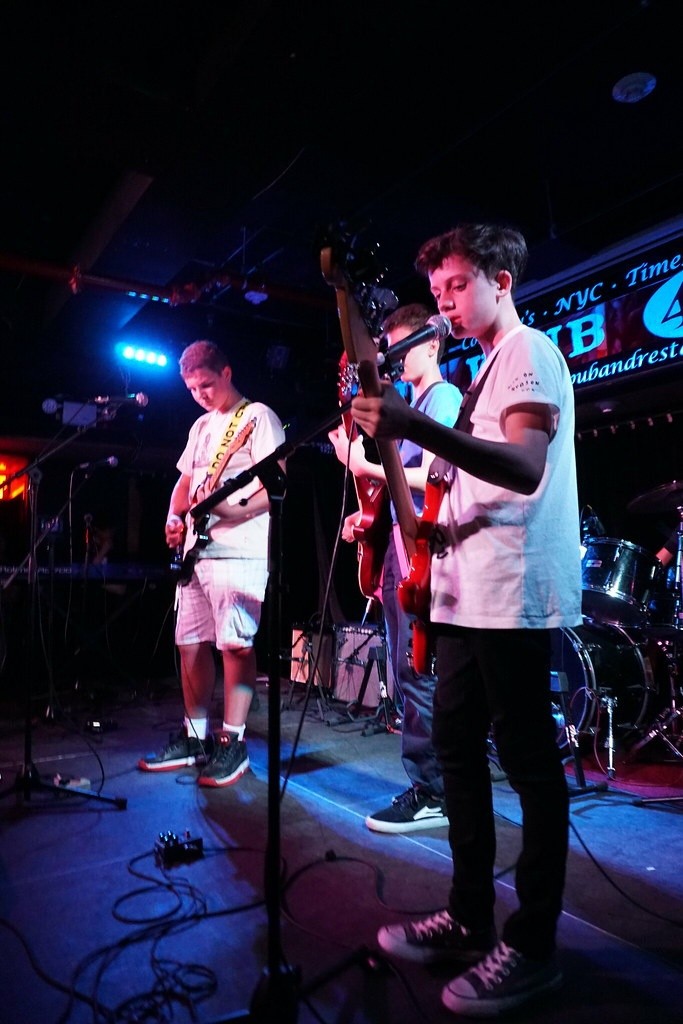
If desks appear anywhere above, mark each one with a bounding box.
[292,628,390,708]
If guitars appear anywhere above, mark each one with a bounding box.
[166,441,296,573]
[321,241,445,674]
[326,349,385,598]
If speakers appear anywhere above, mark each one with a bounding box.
[333,622,395,712]
[290,623,338,696]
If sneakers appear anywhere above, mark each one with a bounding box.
[139,725,212,772]
[377,910,495,964]
[441,941,563,1016]
[197,731,249,788]
[366,783,450,834]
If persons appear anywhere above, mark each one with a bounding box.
[353,221,585,1016]
[138,339,286,792]
[329,305,465,833]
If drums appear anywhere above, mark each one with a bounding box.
[583,536,683,638]
[552,623,646,752]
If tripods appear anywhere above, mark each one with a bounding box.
[0,401,128,808]
[192,364,407,1024]
[618,652,683,766]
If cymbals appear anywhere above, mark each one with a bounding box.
[622,483,682,516]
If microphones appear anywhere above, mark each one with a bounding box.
[76,455,118,469]
[95,392,148,407]
[376,314,452,367]
[83,514,93,529]
[334,657,368,668]
[586,505,606,536]
[279,656,302,662]
